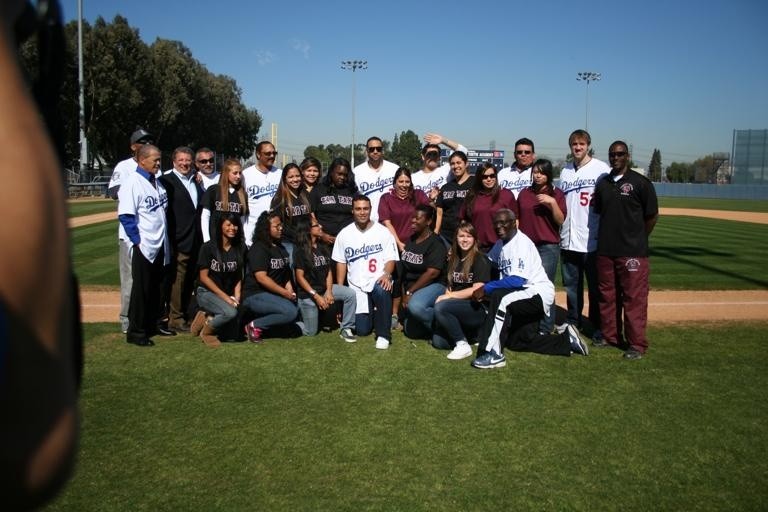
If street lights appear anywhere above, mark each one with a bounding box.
[338,59,368,172]
[576,71,602,134]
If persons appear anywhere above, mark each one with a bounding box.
[106,128,659,369]
[0,30,85,512]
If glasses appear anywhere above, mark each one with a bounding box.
[272,223,282,231]
[609,151,625,156]
[483,173,496,178]
[261,152,278,156]
[198,157,214,164]
[136,139,155,145]
[516,150,530,154]
[368,147,383,153]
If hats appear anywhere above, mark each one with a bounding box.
[130,130,152,144]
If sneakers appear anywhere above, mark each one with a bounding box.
[471,348,506,369]
[244,321,264,345]
[390,316,401,329]
[555,322,588,356]
[376,335,389,349]
[592,338,618,347]
[447,340,473,359]
[623,347,643,360]
[340,328,357,343]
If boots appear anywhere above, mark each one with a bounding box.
[190,310,221,347]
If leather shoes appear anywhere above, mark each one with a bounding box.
[127,336,154,346]
[150,324,189,337]
